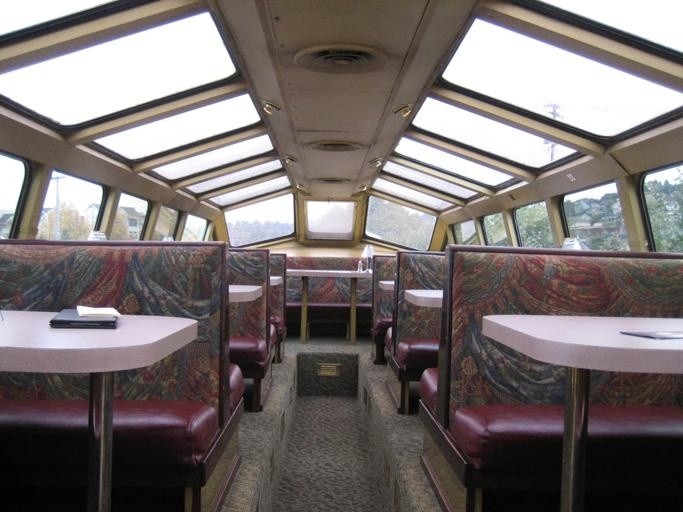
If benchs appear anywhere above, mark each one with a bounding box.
[419,246,682,512]
[1,244,227,512]
[286,256,372,331]
[385,251,447,415]
[371,245,397,369]
[225,249,273,410]
[267,253,286,363]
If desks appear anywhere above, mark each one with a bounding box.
[285,268,372,343]
